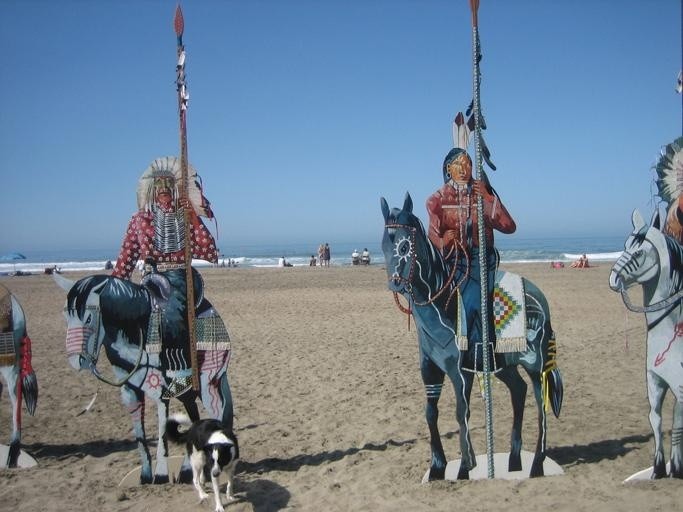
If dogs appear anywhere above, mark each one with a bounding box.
[162,410,240,512]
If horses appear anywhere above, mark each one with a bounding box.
[49,264,236,487]
[376,188,565,484]
[605,207,682,482]
[0,281,40,471]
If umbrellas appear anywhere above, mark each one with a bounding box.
[0,252,27,270]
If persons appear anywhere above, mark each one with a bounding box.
[569,257,582,267]
[109,155,216,401]
[426,148,517,372]
[576,253,589,268]
[103,243,368,269]
